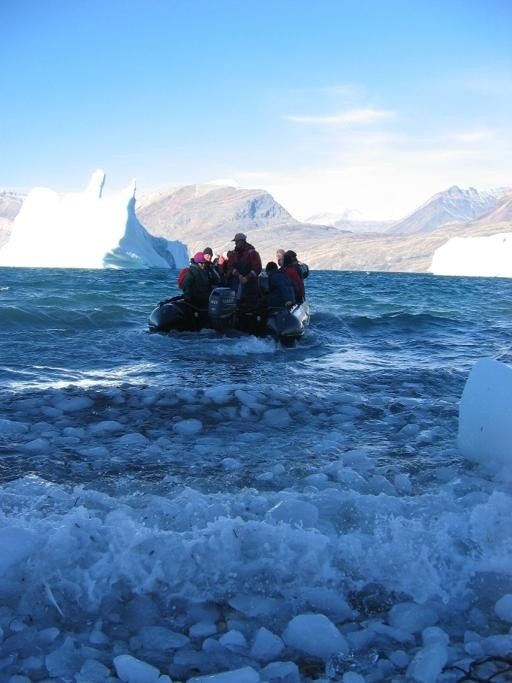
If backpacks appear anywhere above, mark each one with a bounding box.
[178,268,190,289]
[288,261,310,279]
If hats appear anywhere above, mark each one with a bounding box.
[193,251,206,263]
[203,247,214,256]
[231,233,246,241]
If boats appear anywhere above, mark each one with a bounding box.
[147,284,313,349]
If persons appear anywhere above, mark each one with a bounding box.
[178,231,311,316]
